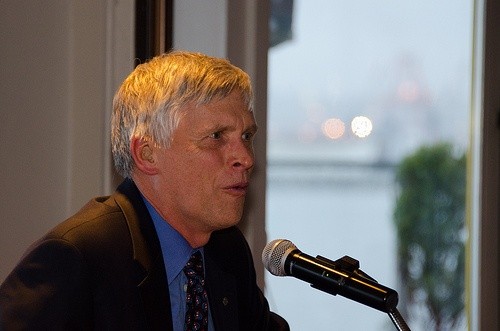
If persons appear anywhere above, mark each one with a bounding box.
[0,49,291,330]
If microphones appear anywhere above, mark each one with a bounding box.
[261,239,398,313]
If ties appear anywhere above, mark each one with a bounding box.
[182,252,210,330]
